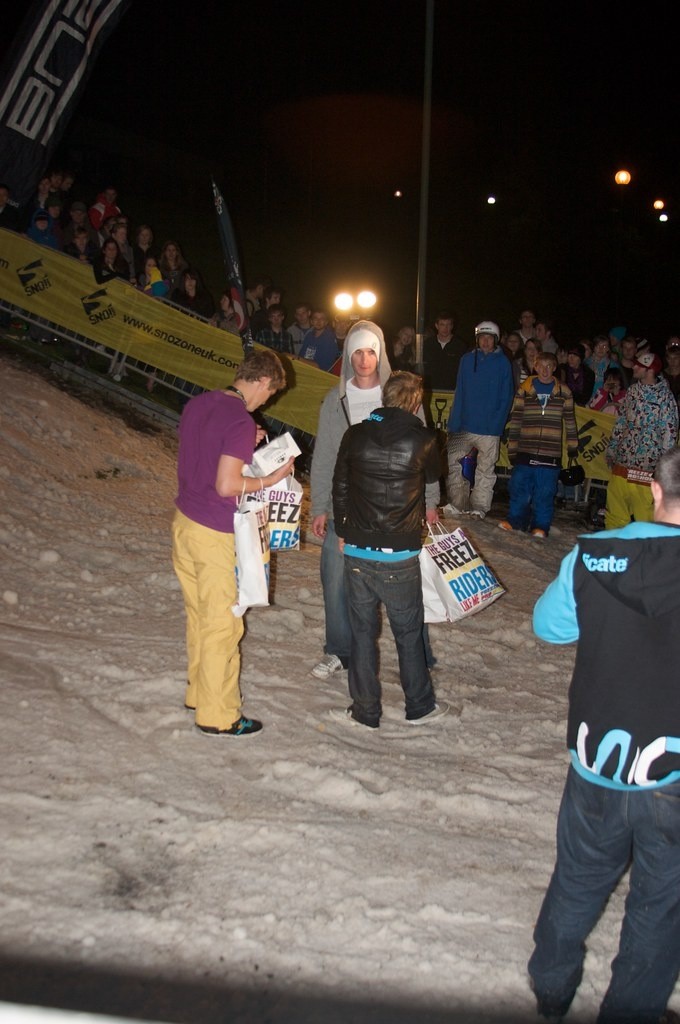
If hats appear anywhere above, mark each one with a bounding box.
[35,211,49,221]
[635,338,651,350]
[72,202,87,212]
[568,343,585,361]
[631,353,662,373]
[347,330,378,364]
[610,327,625,340]
[49,198,62,206]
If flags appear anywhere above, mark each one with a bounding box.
[211,183,255,361]
[0,0,122,208]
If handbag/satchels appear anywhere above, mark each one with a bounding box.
[560,458,585,486]
[418,518,450,621]
[265,467,304,551]
[233,478,269,607]
[426,520,507,623]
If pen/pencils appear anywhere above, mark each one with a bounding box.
[266,434,269,445]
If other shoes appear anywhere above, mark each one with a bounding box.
[597,509,607,516]
[407,701,460,725]
[438,503,470,515]
[329,707,379,730]
[498,520,514,532]
[468,511,486,520]
[531,529,546,539]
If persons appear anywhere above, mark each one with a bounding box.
[172,352,294,734]
[0,171,680,532]
[333,369,450,727]
[498,353,579,539]
[311,319,440,687]
[528,444,680,1024]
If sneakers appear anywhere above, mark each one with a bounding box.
[195,713,263,737]
[309,654,348,679]
[185,694,244,711]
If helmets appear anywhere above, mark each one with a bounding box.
[475,321,500,345]
